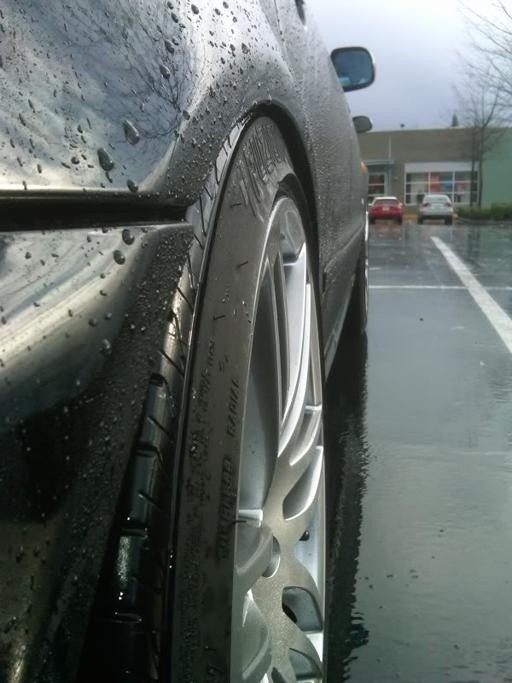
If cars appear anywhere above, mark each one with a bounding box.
[3,0,419,680]
[415,191,454,223]
[367,194,406,225]
